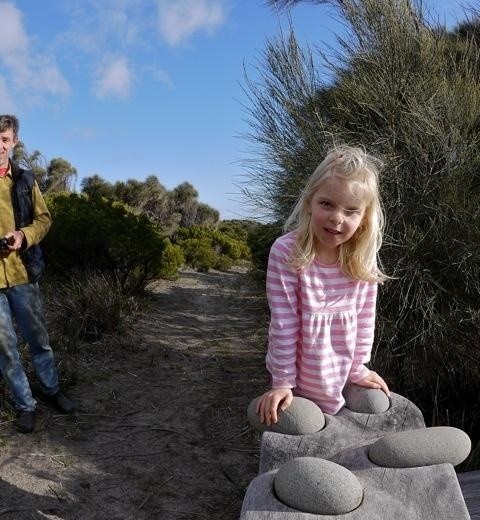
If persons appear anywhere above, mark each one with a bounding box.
[256,130,403,427]
[0,114,73,433]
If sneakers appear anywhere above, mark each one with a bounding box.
[33,385,74,413]
[15,411,35,433]
